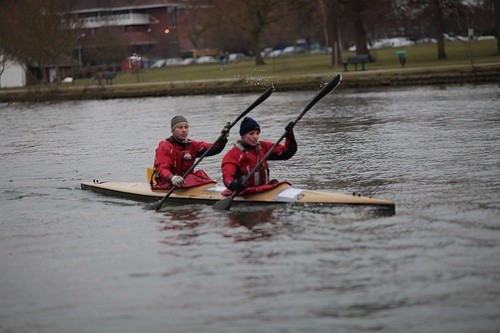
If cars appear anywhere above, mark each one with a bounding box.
[148,32,496,70]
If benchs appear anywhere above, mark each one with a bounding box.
[96,71,117,85]
[342,54,369,71]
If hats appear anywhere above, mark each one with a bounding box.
[171,116,188,131]
[240,117,260,136]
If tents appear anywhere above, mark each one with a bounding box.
[121,33,471,70]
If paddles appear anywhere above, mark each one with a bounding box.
[212,68,342,211]
[139,84,274,210]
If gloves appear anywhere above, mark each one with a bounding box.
[221,122,231,139]
[284,122,295,140]
[233,184,244,194]
[170,175,186,187]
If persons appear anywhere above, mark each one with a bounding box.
[151,115,231,190]
[222,116,298,193]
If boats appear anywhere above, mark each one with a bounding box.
[79,178,396,214]
